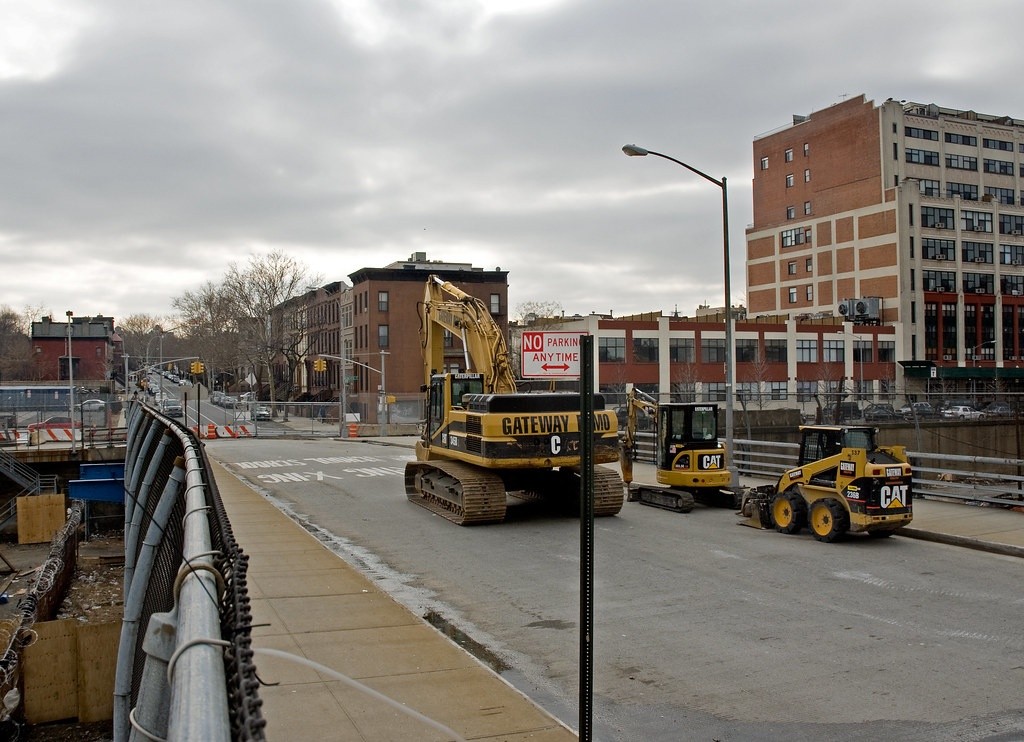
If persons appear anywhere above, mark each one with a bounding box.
[130,391,138,410]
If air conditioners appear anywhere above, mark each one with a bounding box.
[1012,290,1021,296]
[1009,356,1017,360]
[974,356,981,360]
[1011,230,1021,235]
[974,226,984,231]
[975,288,985,293]
[936,255,945,259]
[943,355,952,360]
[937,287,944,291]
[1012,260,1022,265]
[1019,356,1024,360]
[975,258,984,262]
[935,223,944,229]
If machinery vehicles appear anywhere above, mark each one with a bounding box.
[403,272,625,526]
[733,424,915,544]
[616,386,746,514]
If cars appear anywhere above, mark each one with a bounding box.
[862,398,1018,422]
[210,391,256,409]
[249,406,271,422]
[70,398,106,412]
[147,367,193,417]
[28,416,81,429]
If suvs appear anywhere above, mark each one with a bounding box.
[823,401,862,423]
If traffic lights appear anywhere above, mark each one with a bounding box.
[320,359,327,372]
[313,359,321,371]
[190,362,198,373]
[197,362,204,374]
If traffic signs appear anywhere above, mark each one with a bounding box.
[931,367,937,378]
[520,330,590,381]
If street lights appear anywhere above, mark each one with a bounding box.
[621,143,740,488]
[306,285,349,439]
[836,330,865,418]
[65,310,77,453]
[145,334,165,377]
[971,340,997,407]
